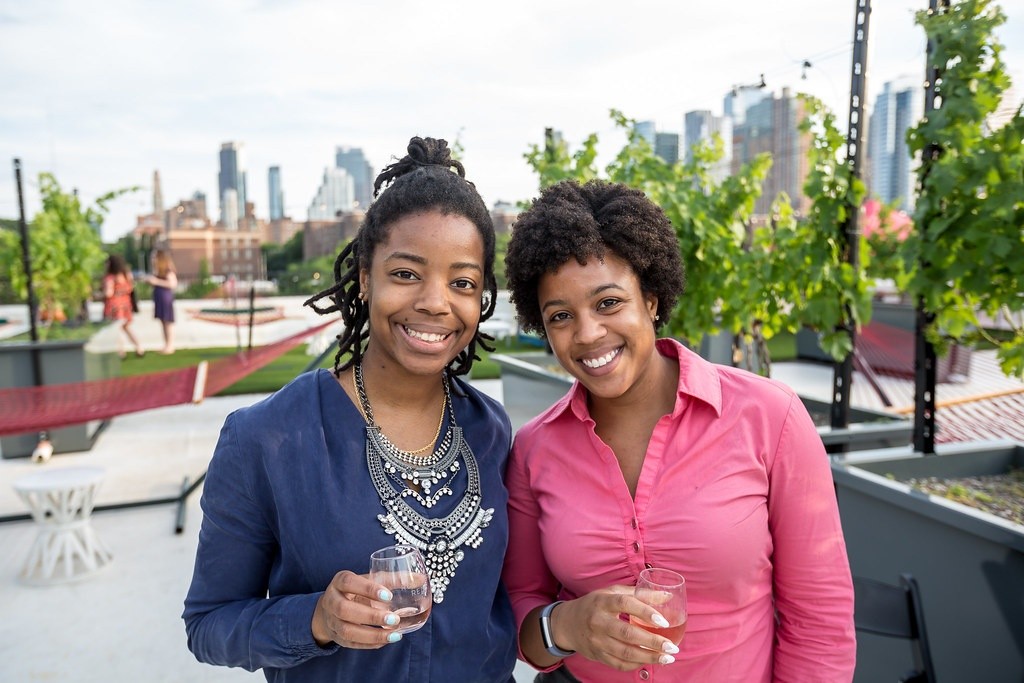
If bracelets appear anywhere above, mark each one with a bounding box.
[539,601,576,658]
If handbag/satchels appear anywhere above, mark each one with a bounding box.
[130,288,139,312]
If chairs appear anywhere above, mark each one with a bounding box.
[530,564,937,683]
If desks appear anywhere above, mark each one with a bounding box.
[10,465,113,588]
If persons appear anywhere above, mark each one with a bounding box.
[352,357,488,605]
[92,255,145,360]
[140,248,178,355]
[182,136,518,683]
[503,179,858,683]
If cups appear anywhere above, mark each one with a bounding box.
[627,568,688,656]
[368,544,431,635]
